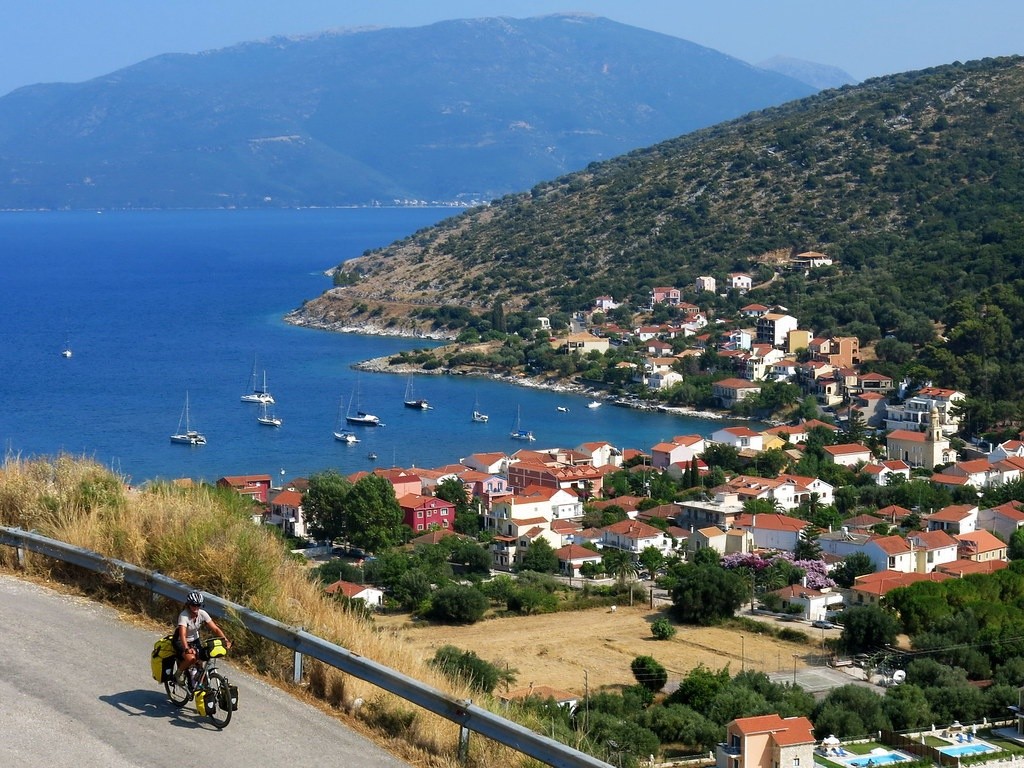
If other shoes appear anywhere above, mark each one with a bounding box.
[171,675,185,686]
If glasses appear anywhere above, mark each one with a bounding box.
[190,603,202,607]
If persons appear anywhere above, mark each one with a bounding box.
[173,592,231,690]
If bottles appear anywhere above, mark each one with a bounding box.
[190,669,196,686]
[194,667,205,682]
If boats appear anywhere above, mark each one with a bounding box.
[584,402,602,409]
[557,406,570,412]
[471,391,488,422]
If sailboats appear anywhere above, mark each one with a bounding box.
[169,390,207,445]
[256,370,281,426]
[345,366,381,426]
[403,366,429,409]
[332,393,357,442]
[508,404,533,440]
[241,351,276,404]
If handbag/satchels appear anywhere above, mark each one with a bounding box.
[218,682,238,711]
[151,639,176,682]
[194,686,216,716]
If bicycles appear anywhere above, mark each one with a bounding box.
[159,636,234,728]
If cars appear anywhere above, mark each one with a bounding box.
[813,620,833,629]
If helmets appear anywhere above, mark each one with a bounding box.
[186,592,203,604]
